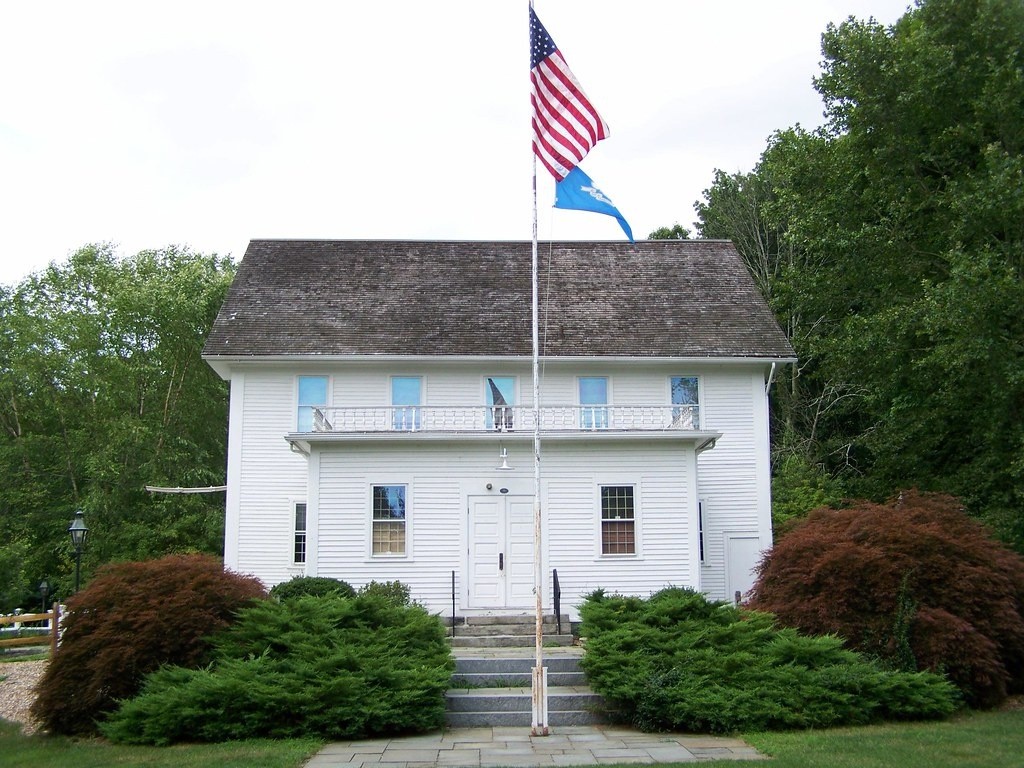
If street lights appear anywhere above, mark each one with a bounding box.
[67,508,90,593]
[38,580,51,627]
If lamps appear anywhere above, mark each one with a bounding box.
[494,442,514,470]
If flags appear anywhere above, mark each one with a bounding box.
[488,378,514,429]
[529,2,611,182]
[554,165,635,244]
